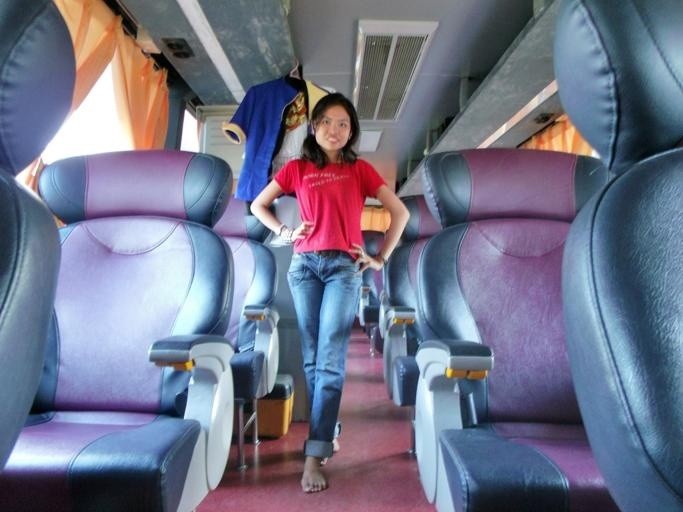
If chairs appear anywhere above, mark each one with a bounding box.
[360,149,614,511]
[0,149,280,512]
[554,1,682,510]
[1,4,77,468]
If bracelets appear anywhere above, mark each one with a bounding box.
[277,224,297,242]
[377,251,389,266]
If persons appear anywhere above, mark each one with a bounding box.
[249,90,412,493]
